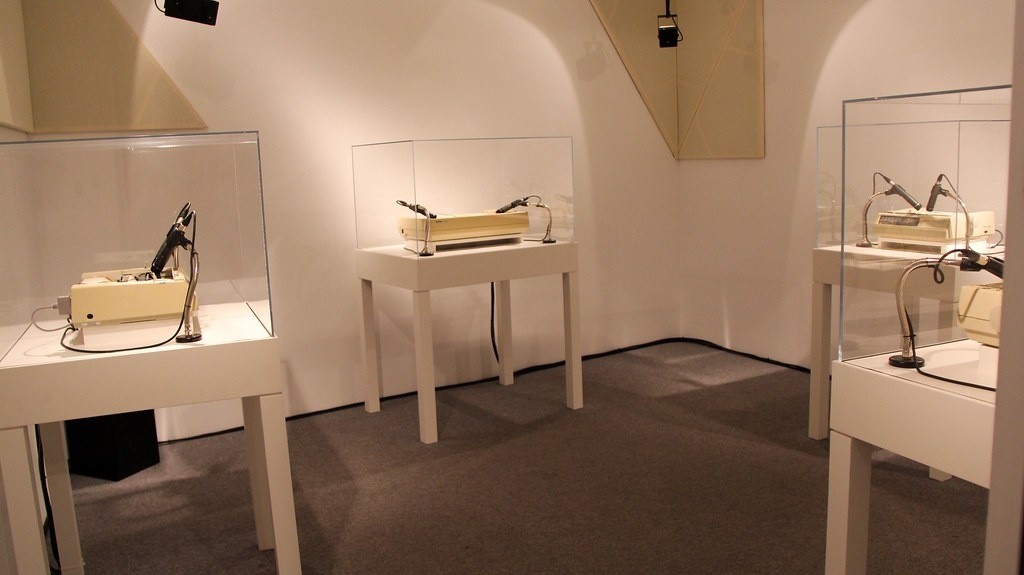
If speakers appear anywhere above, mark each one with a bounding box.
[64,409,161,483]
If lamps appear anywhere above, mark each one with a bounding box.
[656,0,684,48]
[155,0,219,26]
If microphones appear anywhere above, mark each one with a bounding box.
[149,207,193,279]
[884,176,922,211]
[400,200,437,219]
[960,248,1005,279]
[926,175,943,211]
[496,196,530,213]
